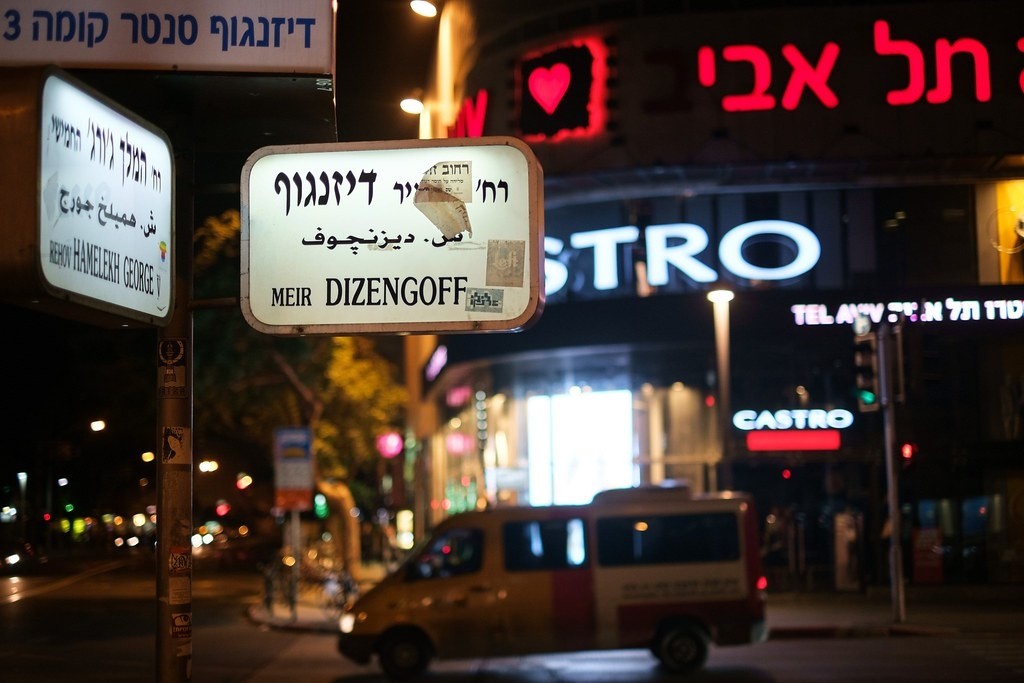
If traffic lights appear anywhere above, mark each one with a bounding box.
[853,332,878,412]
[780,466,792,479]
[900,443,913,462]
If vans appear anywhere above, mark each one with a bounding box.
[337,481,770,677]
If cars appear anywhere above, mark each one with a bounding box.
[0,543,31,574]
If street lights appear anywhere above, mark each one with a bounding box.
[705,284,737,491]
